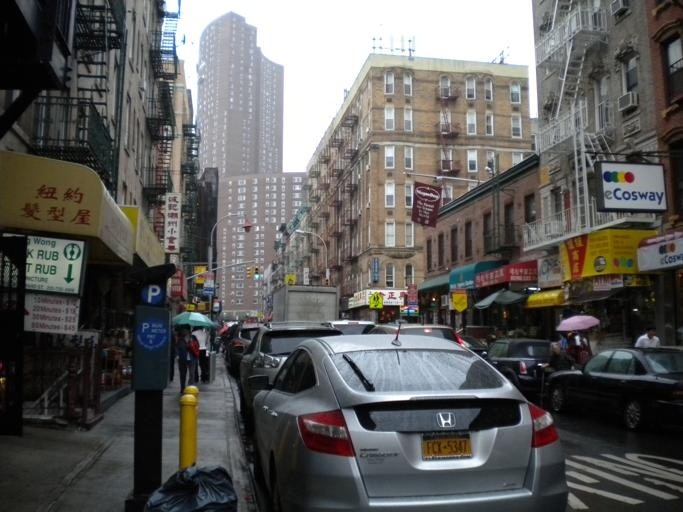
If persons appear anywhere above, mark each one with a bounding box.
[210,328,216,352]
[634,327,660,348]
[191,326,208,382]
[178,325,200,393]
[558,331,588,371]
[221,333,227,354]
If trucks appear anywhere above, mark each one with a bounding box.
[271,281,341,324]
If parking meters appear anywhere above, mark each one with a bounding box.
[124,263,176,511]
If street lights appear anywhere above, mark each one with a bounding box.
[207,212,250,316]
[295,230,330,281]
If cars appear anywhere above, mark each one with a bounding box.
[550,346,683,431]
[254,330,566,510]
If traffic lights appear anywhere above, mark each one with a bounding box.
[246,266,264,281]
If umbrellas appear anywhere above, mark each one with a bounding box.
[219,326,229,336]
[557,315,600,331]
[170,312,214,328]
